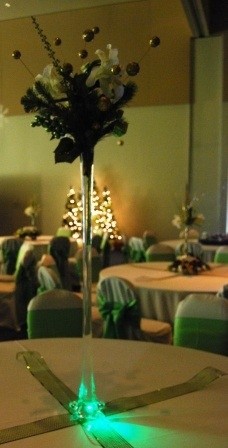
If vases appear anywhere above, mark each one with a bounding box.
[66,150,105,423]
[169,256,209,275]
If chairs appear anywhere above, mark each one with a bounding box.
[40,255,97,305]
[75,247,101,281]
[0,237,31,282]
[171,293,228,357]
[174,242,206,258]
[144,229,157,249]
[58,227,72,236]
[144,244,174,260]
[97,276,173,343]
[26,290,82,337]
[0,245,38,331]
[37,267,101,321]
[213,246,228,263]
[126,236,147,261]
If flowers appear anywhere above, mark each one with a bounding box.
[173,194,204,255]
[11,15,159,245]
